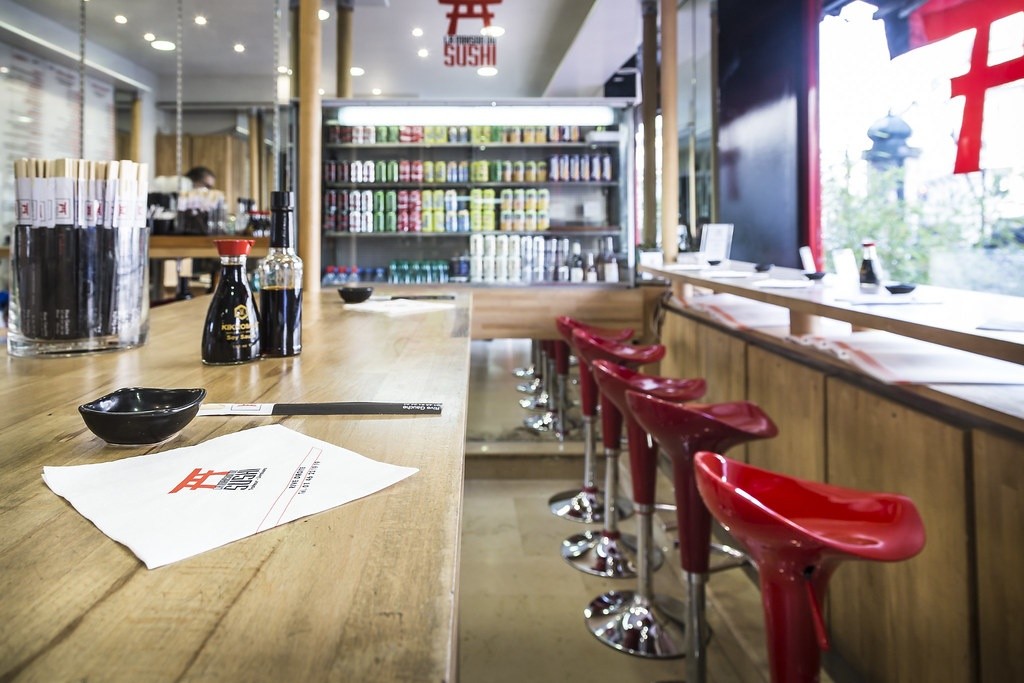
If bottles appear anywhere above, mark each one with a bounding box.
[570,240,584,282]
[604,236,619,283]
[585,253,597,282]
[860,242,879,295]
[596,239,604,280]
[260,191,303,356]
[321,257,450,287]
[202,239,262,364]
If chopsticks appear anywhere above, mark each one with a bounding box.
[369,295,455,300]
[200,401,443,417]
[14,159,148,225]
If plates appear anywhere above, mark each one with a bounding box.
[886,285,914,293]
[708,261,721,265]
[755,264,772,272]
[805,273,826,279]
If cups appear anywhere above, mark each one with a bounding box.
[7,227,150,357]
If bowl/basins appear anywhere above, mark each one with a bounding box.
[338,286,372,303]
[78,388,206,445]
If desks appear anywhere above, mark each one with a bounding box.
[1,287,475,683]
[147,235,271,293]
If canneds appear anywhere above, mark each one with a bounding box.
[322,126,612,283]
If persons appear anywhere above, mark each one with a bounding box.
[183,167,216,189]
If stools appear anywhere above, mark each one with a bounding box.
[562,328,665,580]
[548,317,637,524]
[583,359,712,660]
[513,338,585,432]
[625,389,779,683]
[695,450,925,683]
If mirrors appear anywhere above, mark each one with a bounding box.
[468,338,640,443]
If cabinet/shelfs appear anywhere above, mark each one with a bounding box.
[289,99,668,481]
